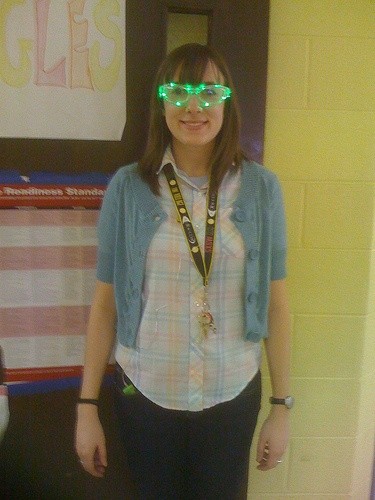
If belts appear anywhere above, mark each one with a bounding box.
[116,361,133,385]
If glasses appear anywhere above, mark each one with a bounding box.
[158,82,231,106]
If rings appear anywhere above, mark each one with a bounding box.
[78,459,84,465]
[276,459,283,463]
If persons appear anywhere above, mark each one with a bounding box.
[75,43,293,500]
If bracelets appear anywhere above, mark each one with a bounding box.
[77,397,102,407]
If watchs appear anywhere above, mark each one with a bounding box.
[269,395,293,411]
